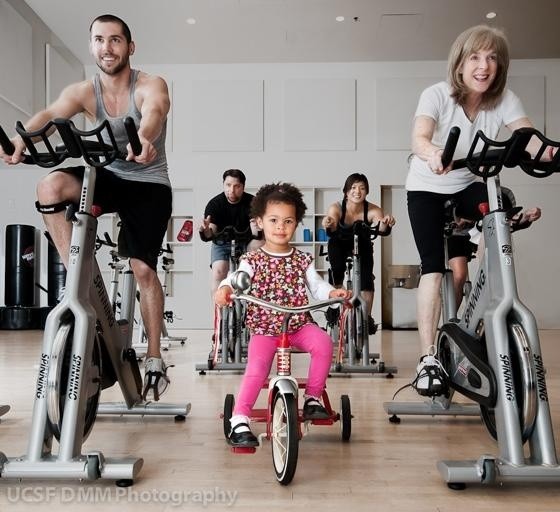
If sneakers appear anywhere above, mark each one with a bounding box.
[302,396,329,422]
[326,306,340,322]
[368,315,377,335]
[142,357,171,401]
[229,422,260,448]
[415,355,447,393]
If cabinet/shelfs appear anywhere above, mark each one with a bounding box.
[245,185,343,284]
[94,189,195,330]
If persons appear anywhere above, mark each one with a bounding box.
[213,181,354,447]
[322,172,396,336]
[447,207,541,312]
[405,25,559,396]
[200,169,263,342]
[1,14,174,400]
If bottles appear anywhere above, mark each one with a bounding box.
[318,229,325,241]
[304,229,310,242]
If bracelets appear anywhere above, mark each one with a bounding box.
[549,146,555,161]
